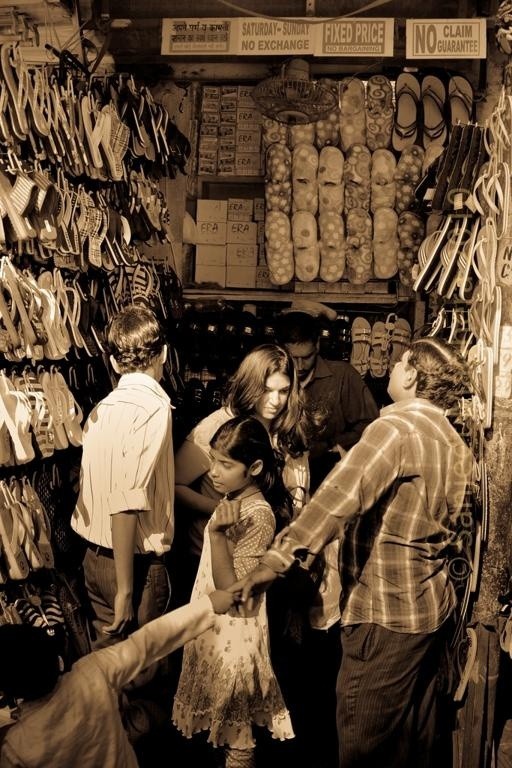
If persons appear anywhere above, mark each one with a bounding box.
[174,340,333,560]
[228,334,480,768]
[170,417,297,768]
[273,311,380,498]
[0,589,241,768]
[67,303,177,738]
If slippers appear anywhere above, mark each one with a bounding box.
[439,460,488,702]
[261,0,512,429]
[1,29,184,657]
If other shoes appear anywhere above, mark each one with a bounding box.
[184,302,260,441]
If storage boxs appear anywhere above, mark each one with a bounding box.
[180,84,271,294]
